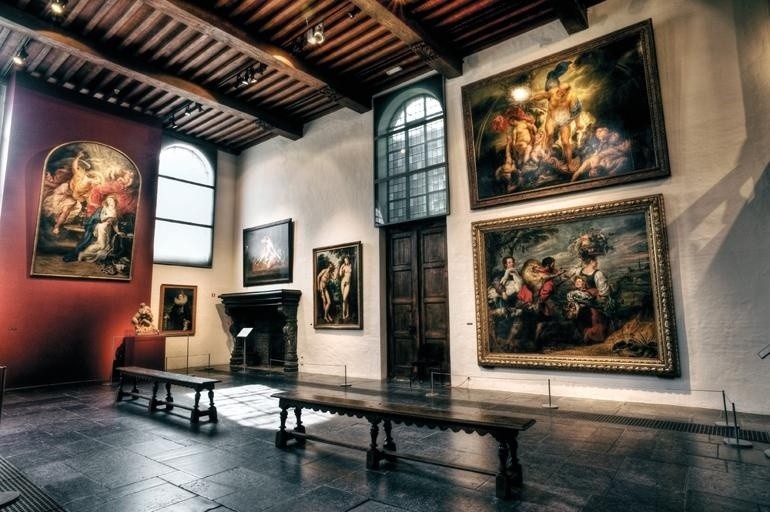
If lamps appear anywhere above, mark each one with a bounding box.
[13,45,28,65]
[51,0,68,13]
[307,22,325,45]
[169,102,204,129]
[232,62,264,89]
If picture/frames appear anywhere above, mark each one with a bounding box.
[312,240,363,330]
[461,18,671,213]
[30,140,142,282]
[158,284,197,337]
[472,193,681,379]
[242,218,294,287]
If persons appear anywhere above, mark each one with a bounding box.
[487,248,614,354]
[38,143,140,274]
[163,290,192,331]
[132,302,160,335]
[316,256,354,324]
[494,61,638,195]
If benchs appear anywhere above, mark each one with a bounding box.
[270,390,536,499]
[116,366,222,424]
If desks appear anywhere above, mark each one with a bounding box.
[124,336,166,381]
